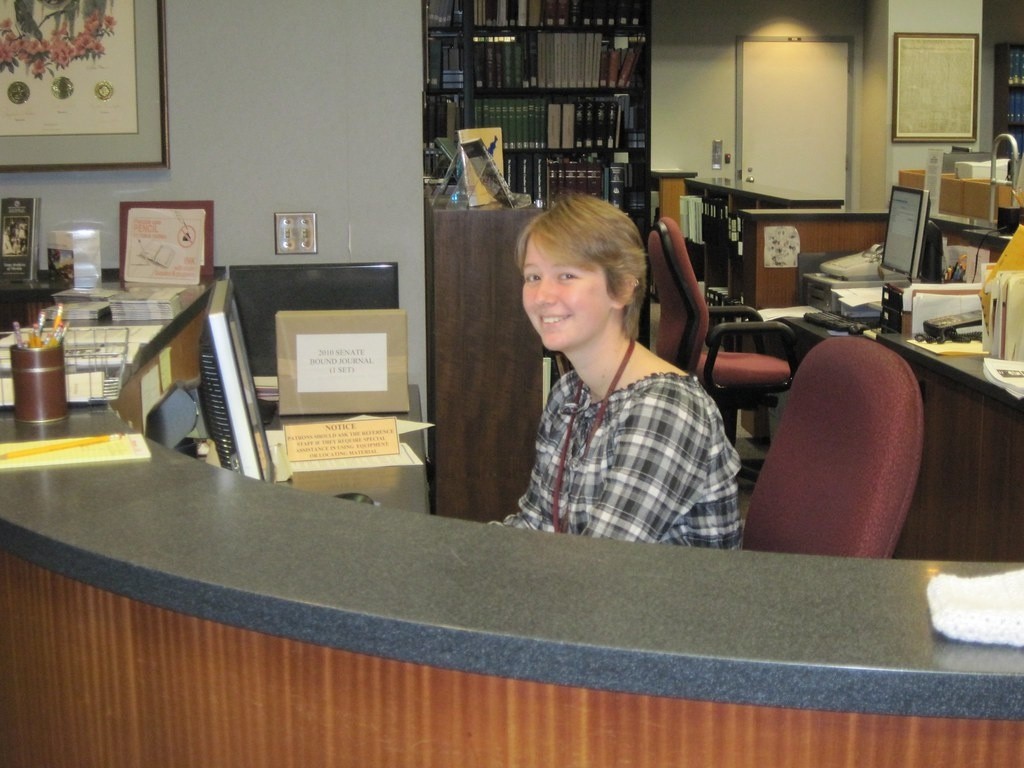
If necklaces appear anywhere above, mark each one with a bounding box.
[553,338,636,533]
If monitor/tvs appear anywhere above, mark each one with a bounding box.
[146,278,276,484]
[879,182,944,288]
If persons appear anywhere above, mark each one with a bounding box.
[482,193,744,552]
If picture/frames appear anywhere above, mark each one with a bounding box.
[891,32,980,144]
[0,0,169,172]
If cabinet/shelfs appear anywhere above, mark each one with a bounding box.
[683,179,844,346]
[993,41,1024,163]
[421,0,651,248]
[423,184,547,519]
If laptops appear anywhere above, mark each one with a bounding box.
[848,323,869,334]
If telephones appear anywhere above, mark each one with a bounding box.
[924,310,983,334]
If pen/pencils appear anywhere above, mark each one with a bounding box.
[0,433,121,461]
[945,255,966,280]
[54,303,63,331]
[38,312,45,335]
[28,322,71,349]
[12,322,23,348]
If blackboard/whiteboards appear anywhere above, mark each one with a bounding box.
[736,36,857,210]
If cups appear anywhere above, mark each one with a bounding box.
[11,344,68,423]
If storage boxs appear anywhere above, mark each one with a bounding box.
[275,309,411,414]
[899,150,1013,222]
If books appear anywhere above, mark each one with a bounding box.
[1009,48,1024,158]
[424,0,639,215]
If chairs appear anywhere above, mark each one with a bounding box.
[648,216,795,484]
[741,336,933,559]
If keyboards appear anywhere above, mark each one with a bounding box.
[804,309,859,332]
